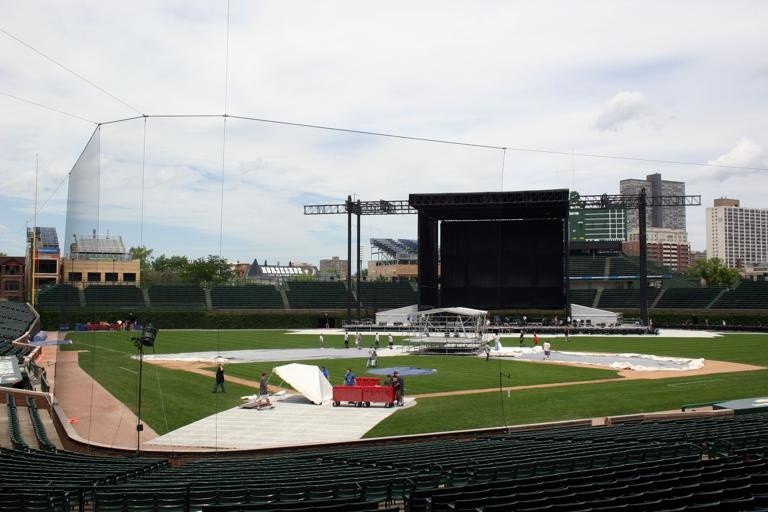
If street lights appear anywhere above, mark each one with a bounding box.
[112,257,115,285]
[71,257,74,284]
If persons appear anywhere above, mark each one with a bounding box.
[315,454,325,463]
[128,313,135,324]
[722,320,727,326]
[255,371,276,410]
[650,317,653,330]
[17,357,28,389]
[12,443,24,456]
[213,364,227,393]
[319,313,571,406]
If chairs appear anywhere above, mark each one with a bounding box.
[6,393,54,452]
[1,408,768,512]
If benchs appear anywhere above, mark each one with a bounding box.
[564,251,764,316]
[1,301,39,364]
[36,278,417,315]
